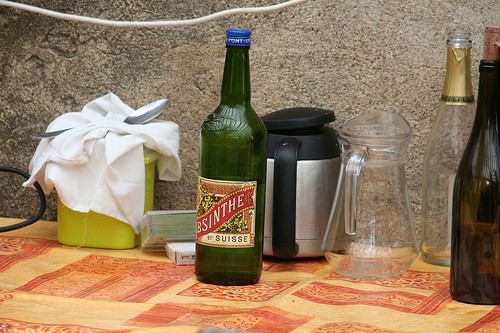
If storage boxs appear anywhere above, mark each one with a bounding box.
[57,146,160,249]
[163,240,195,266]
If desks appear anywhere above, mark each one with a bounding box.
[0,217,500,333]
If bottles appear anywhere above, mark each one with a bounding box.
[450,24,500,307]
[419,27,485,268]
[193,28,270,286]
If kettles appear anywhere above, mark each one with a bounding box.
[261,104,347,261]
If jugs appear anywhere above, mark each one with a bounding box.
[319,111,423,279]
